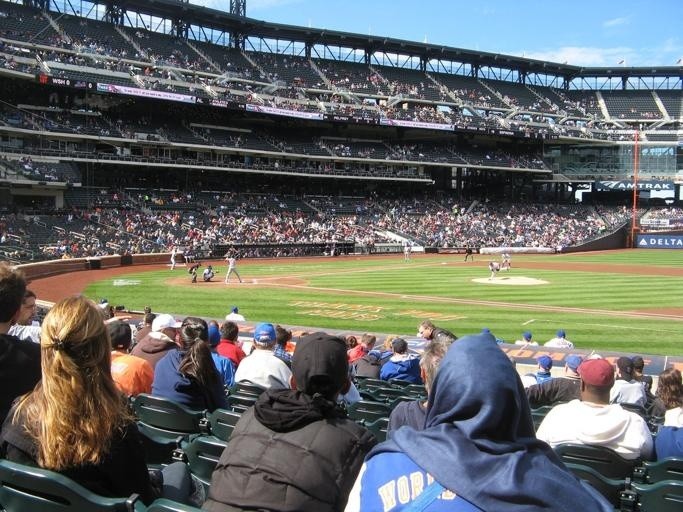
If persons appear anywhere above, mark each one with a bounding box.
[487,262,510,280]
[501,253,510,271]
[223,255,244,285]
[0,5,683,253]
[224,306,246,320]
[463,245,474,262]
[1,255,231,496]
[203,327,682,510]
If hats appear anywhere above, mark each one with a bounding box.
[523,331,532,340]
[535,354,552,370]
[207,324,221,346]
[254,322,276,342]
[565,354,644,387]
[291,332,347,395]
[368,350,381,361]
[557,331,565,337]
[108,320,131,349]
[151,313,183,331]
